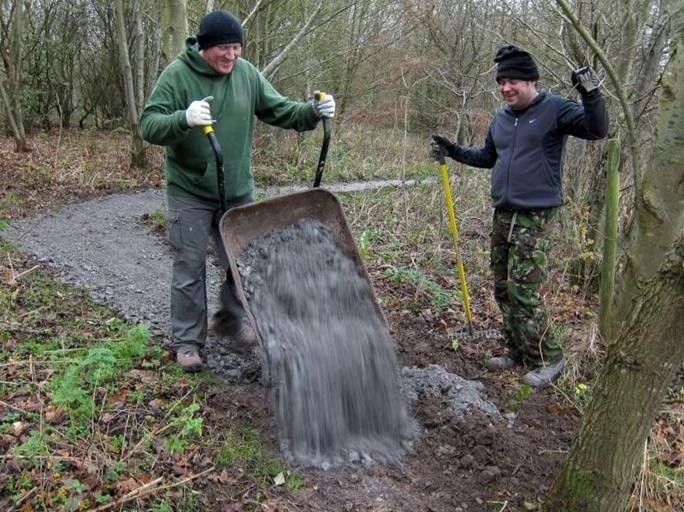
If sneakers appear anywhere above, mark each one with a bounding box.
[488,353,515,373]
[208,312,256,345]
[175,350,202,371]
[520,354,566,390]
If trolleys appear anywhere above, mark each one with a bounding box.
[201,91,390,349]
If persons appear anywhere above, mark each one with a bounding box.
[426,42,611,390]
[139,10,337,371]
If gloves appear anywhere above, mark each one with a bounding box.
[185,94,214,130]
[570,65,597,96]
[430,134,455,162]
[309,88,337,120]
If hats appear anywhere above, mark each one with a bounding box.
[195,9,245,50]
[492,44,540,85]
